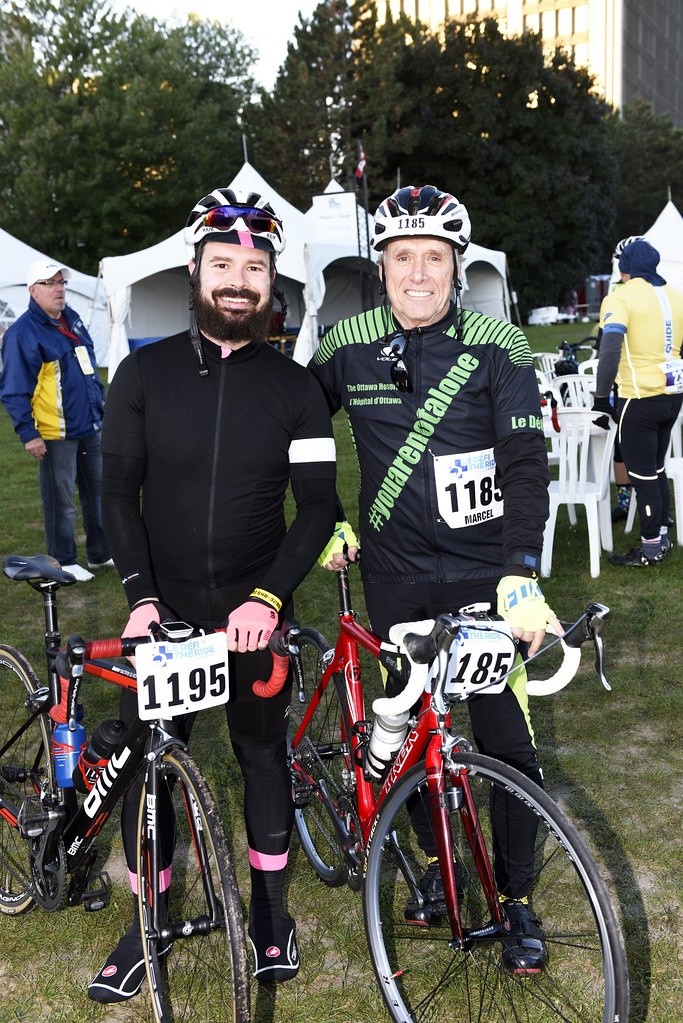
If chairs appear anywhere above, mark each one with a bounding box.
[531,347,683,578]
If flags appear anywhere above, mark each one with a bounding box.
[354,143,368,178]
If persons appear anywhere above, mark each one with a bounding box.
[0,260,115,582]
[590,236,683,568]
[88,188,301,1005]
[304,184,565,976]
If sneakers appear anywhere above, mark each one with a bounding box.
[248,904,299,982]
[405,856,464,926]
[606,535,672,567]
[499,898,550,975]
[611,505,629,522]
[88,556,116,569]
[59,563,96,582]
[87,924,174,1003]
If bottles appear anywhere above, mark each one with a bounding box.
[72,719,127,794]
[53,720,87,787]
[366,709,409,777]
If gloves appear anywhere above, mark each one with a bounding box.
[590,397,621,431]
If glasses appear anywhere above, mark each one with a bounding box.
[196,203,284,234]
[35,279,68,288]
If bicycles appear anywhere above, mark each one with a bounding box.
[284,540,632,1023]
[1,551,253,1023]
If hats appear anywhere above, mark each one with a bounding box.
[26,262,71,289]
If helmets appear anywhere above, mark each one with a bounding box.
[613,237,651,259]
[369,186,472,255]
[185,190,286,255]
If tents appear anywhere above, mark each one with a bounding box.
[0,162,683,384]
